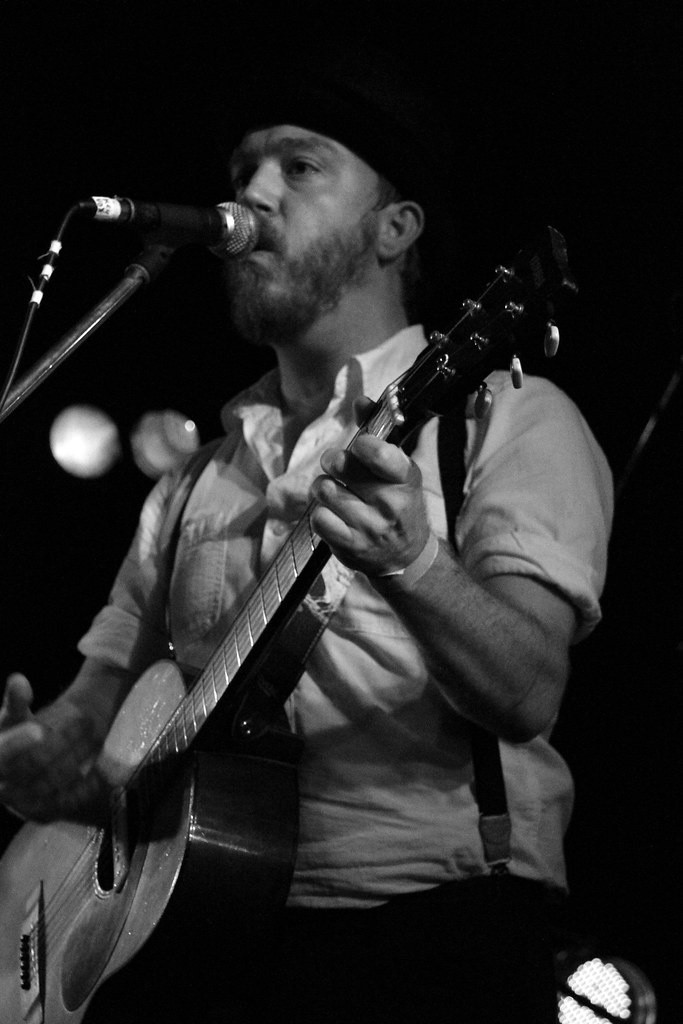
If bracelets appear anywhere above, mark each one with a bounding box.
[363,523,442,601]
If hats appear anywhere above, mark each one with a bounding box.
[217,37,460,269]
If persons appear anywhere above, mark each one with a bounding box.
[1,60,622,1024]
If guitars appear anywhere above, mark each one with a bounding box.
[1,217,580,1023]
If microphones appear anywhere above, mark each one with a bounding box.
[87,195,259,258]
